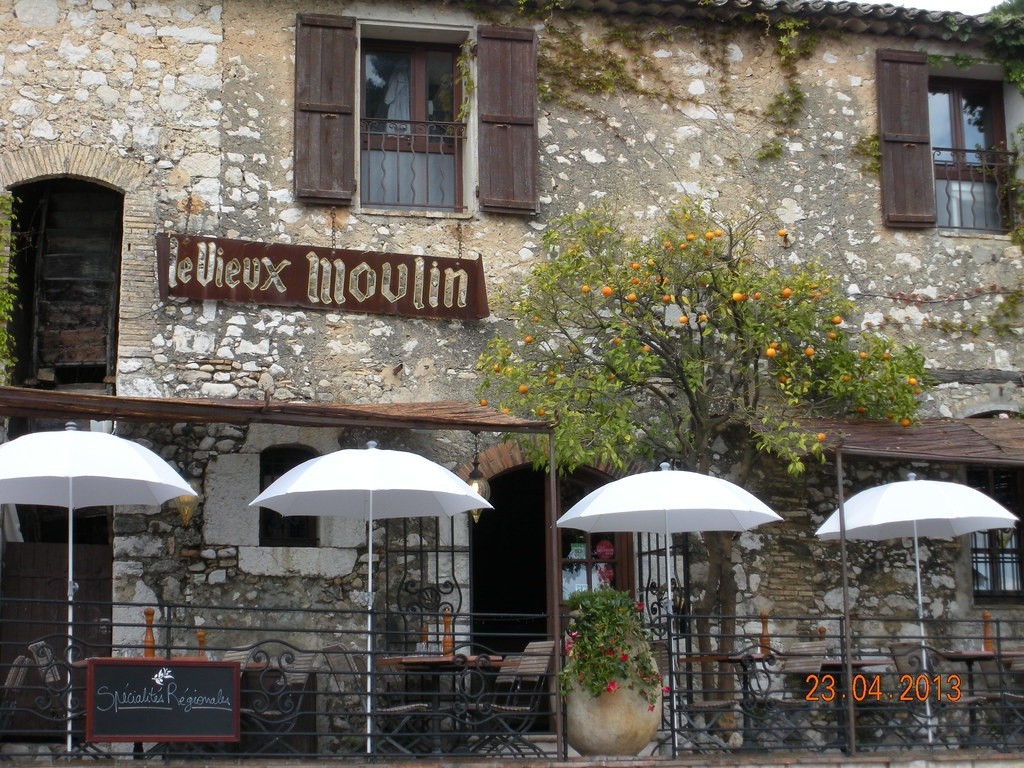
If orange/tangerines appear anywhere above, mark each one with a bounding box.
[481,229,921,439]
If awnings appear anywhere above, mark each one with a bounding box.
[742,415,1024,755]
[1,385,563,762]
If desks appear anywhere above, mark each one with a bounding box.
[931,649,1024,749]
[377,654,502,757]
[678,655,791,754]
[821,660,894,754]
[395,662,522,753]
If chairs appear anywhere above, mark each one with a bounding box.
[0,640,556,759]
[648,641,1023,755]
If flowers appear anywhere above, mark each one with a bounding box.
[557,584,670,712]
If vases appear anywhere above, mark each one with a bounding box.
[565,640,663,759]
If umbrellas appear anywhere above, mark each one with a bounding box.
[551,470,785,756]
[813,480,1020,744]
[0,431,198,753]
[249,449,495,754]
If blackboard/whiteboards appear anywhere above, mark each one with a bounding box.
[86,657,240,742]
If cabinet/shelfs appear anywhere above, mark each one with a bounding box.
[241,669,317,758]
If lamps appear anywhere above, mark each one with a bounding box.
[466,427,492,523]
[171,421,202,529]
